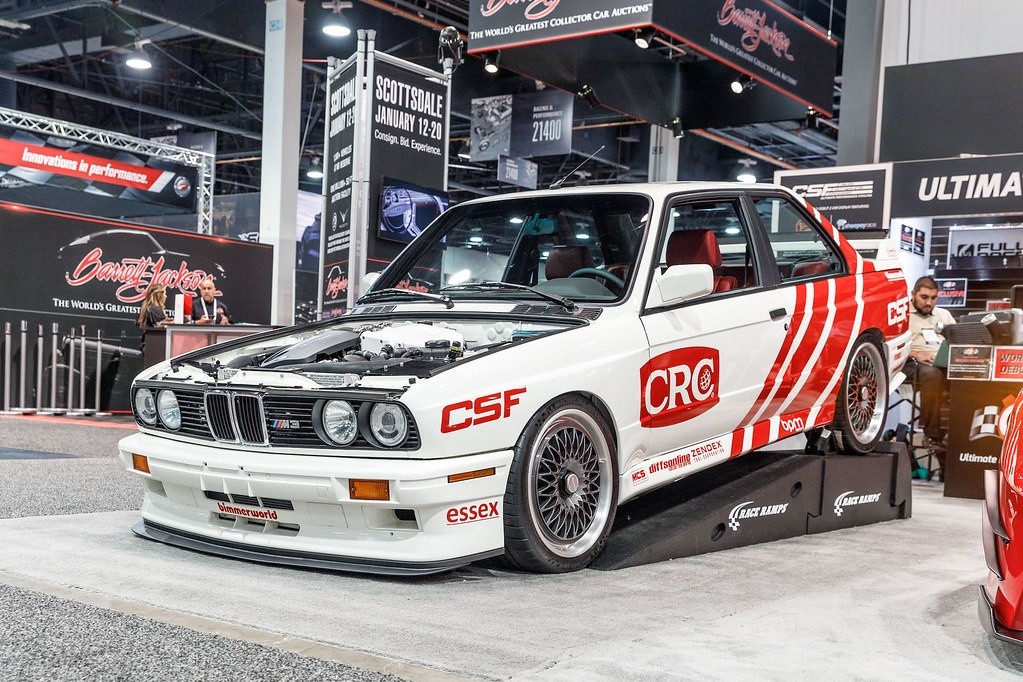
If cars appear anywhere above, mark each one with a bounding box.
[117,181,911,577]
[980,388,1023,646]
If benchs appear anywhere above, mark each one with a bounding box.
[721,260,829,287]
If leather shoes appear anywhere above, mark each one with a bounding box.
[922,436,943,452]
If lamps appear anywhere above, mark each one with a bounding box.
[321,0,354,37]
[730,73,759,94]
[633,25,656,50]
[125,34,154,71]
[806,107,821,129]
[577,84,600,110]
[666,117,684,139]
[484,50,501,74]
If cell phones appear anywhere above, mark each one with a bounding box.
[209,315,215,319]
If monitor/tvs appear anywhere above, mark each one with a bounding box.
[375,177,450,250]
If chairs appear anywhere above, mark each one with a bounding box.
[902,357,951,446]
[545,245,596,284]
[666,228,738,296]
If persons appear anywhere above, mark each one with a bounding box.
[139,283,173,327]
[907,276,956,451]
[185,280,234,325]
